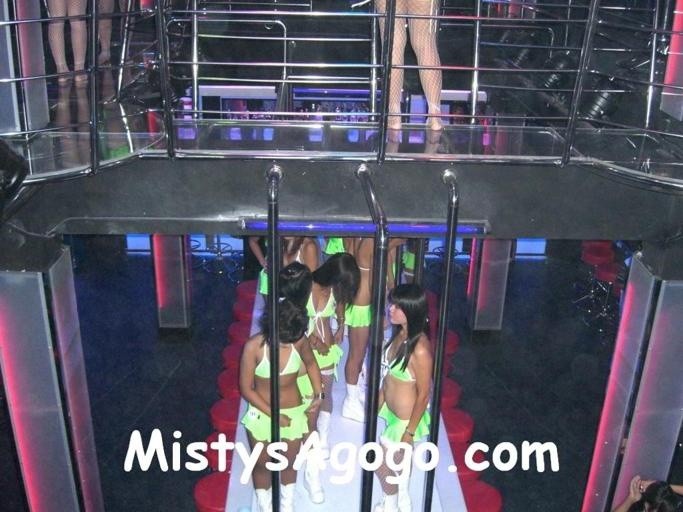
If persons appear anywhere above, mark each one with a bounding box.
[94,1,136,66]
[51,78,92,171]
[247,235,424,507]
[97,57,139,163]
[42,1,89,83]
[371,0,443,130]
[612,476,683,511]
[383,129,443,153]
[238,295,324,512]
[372,282,433,512]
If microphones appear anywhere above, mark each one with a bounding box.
[193,277,259,510]
[568,240,628,333]
[425,286,501,511]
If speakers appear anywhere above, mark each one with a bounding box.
[302,447,323,504]
[376,492,399,512]
[316,410,331,459]
[341,384,366,422]
[359,368,366,404]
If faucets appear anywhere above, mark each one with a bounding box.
[429,118,442,131]
[388,117,401,130]
